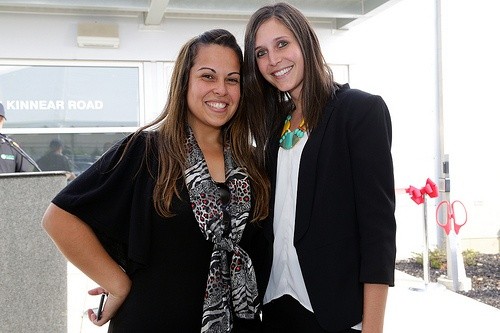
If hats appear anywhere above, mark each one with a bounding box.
[0,103,7,120]
[49,139,64,148]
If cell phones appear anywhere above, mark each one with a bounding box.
[97,293,105,319]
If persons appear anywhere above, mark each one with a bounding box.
[224,2,397,333]
[0,103,27,173]
[33,139,75,172]
[41,29,264,333]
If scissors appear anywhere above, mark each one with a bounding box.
[435,197,468,293]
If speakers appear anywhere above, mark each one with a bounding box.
[76,21,119,47]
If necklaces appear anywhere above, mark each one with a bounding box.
[279,114,310,150]
